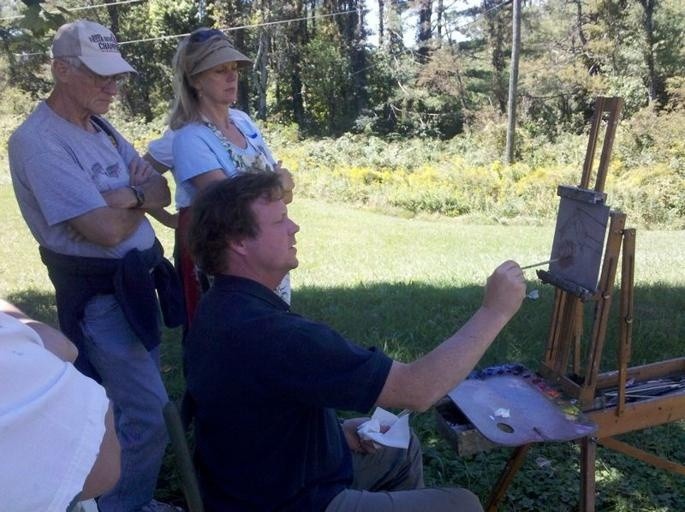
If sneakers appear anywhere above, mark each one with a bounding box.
[134,498,183,511]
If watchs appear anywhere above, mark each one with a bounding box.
[126,184,144,208]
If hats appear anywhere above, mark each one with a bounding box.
[51,20,137,76]
[182,28,253,77]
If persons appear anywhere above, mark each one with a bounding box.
[9,21,171,511]
[182,168,527,511]
[170,28,291,305]
[0,299,121,511]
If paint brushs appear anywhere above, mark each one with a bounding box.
[521,256,569,269]
[596,376,685,404]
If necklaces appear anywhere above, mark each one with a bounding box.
[203,122,267,174]
